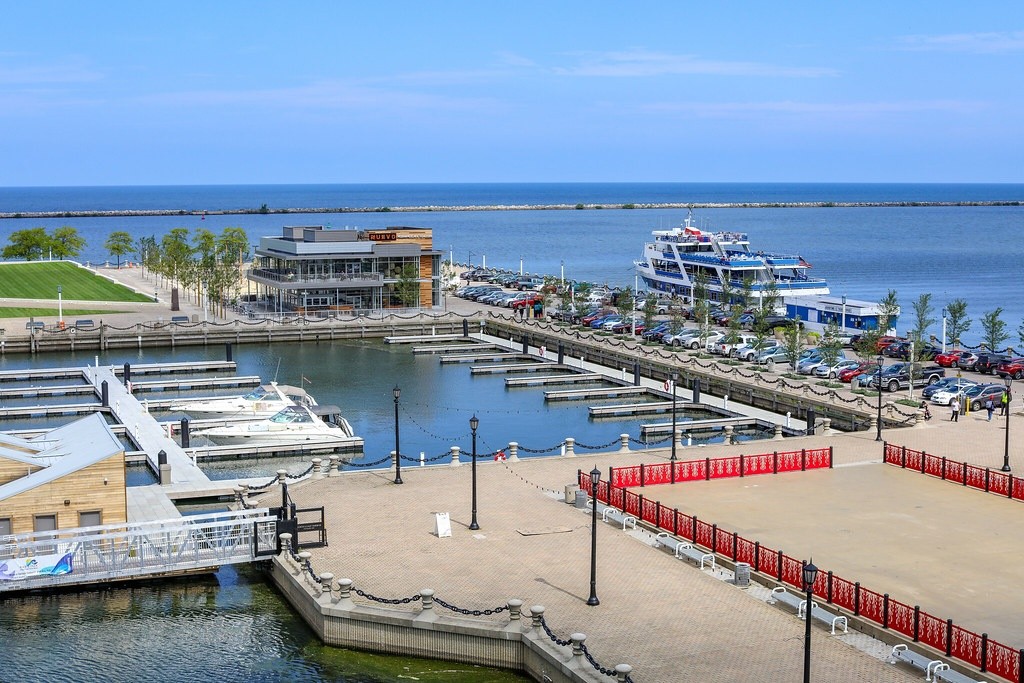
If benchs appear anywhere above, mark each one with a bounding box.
[802,602,848,635]
[586,499,636,531]
[76,320,94,327]
[892,643,943,681]
[26,322,44,329]
[932,663,987,683]
[771,587,807,618]
[172,317,189,323]
[655,532,715,570]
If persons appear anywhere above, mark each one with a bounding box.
[919,401,931,418]
[951,399,961,422]
[999,389,1010,416]
[986,395,994,422]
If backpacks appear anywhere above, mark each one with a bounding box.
[985,400,992,408]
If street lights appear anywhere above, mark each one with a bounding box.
[519,254,524,275]
[469,412,480,530]
[202,280,208,321]
[941,308,948,353]
[56,285,63,328]
[874,356,885,442]
[841,293,848,332]
[802,558,818,683]
[560,259,565,285]
[586,464,601,605]
[1000,373,1012,471]
[392,383,403,484]
[670,366,680,461]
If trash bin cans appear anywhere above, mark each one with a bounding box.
[768,362,776,373]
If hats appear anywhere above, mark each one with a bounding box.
[989,395,994,398]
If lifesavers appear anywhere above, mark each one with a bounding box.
[171,425,174,435]
[494,452,506,462]
[357,319,362,324]
[130,382,133,393]
[538,347,543,356]
[70,327,76,334]
[303,318,311,326]
[664,382,669,391]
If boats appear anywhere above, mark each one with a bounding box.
[189,373,354,446]
[631,209,901,337]
[167,355,319,421]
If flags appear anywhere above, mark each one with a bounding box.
[798,256,812,268]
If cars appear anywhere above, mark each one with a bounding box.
[452,266,1024,412]
